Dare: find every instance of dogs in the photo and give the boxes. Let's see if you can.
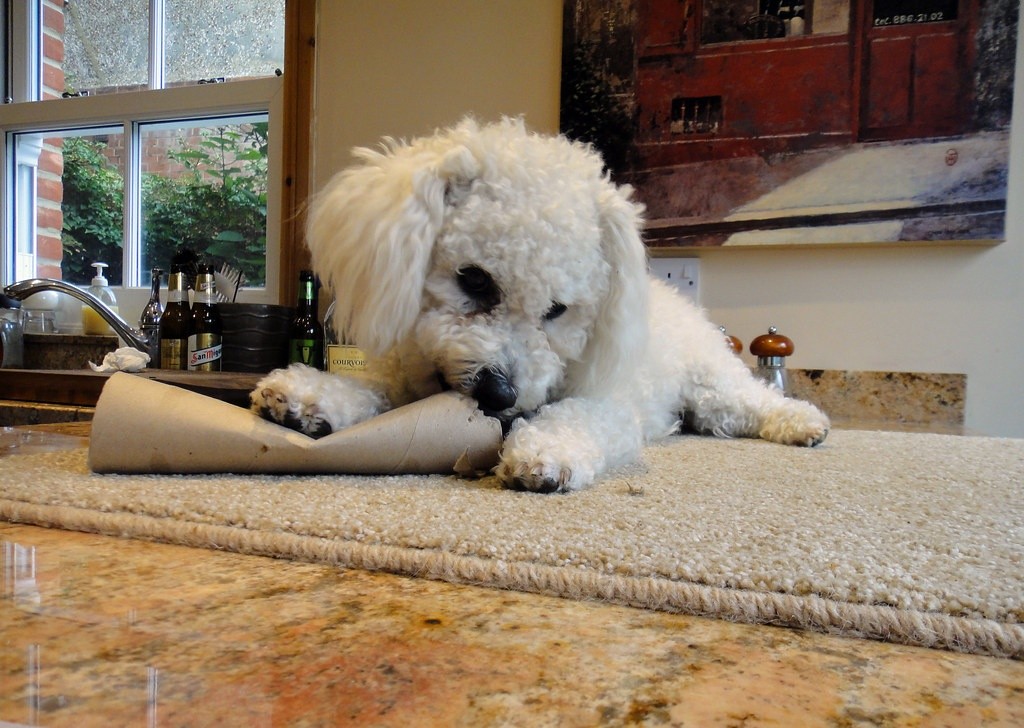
[249,112,828,493]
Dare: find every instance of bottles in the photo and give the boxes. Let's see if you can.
[160,264,190,373]
[720,326,742,356]
[187,265,223,372]
[747,327,794,391]
[292,271,322,368]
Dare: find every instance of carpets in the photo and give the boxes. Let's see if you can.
[0,428,1024,662]
